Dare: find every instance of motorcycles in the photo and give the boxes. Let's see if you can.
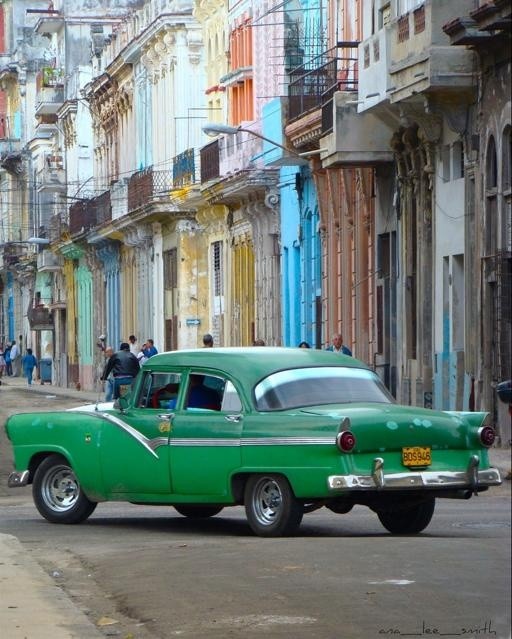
[99,371,139,401]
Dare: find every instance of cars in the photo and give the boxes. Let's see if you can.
[1,344,502,537]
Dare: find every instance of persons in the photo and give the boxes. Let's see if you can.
[299,342,309,348]
[254,340,265,346]
[99,336,158,402]
[167,374,220,409]
[326,333,352,355]
[20,348,38,387]
[203,334,213,348]
[0,335,23,385]
